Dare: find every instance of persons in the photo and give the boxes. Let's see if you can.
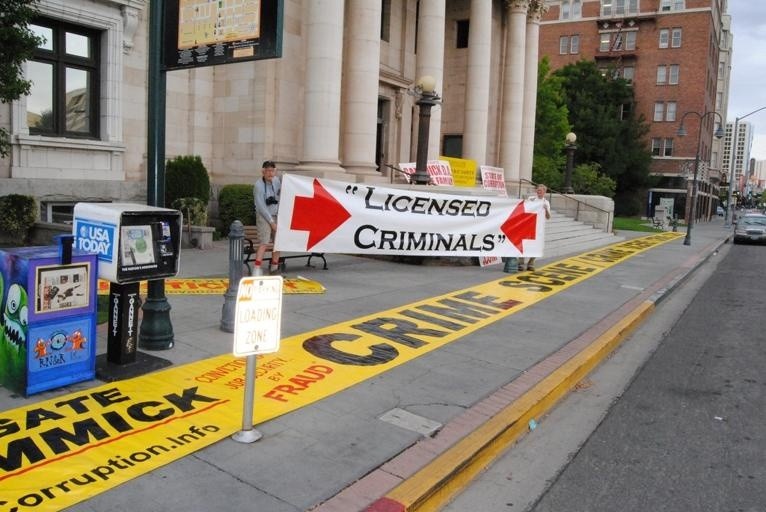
[518,184,551,270]
[252,160,282,278]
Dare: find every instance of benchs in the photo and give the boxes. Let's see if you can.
[241,223,328,277]
[651,216,665,231]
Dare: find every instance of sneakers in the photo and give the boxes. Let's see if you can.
[518,262,535,271]
[269,267,288,278]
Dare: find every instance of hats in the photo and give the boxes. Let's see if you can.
[262,160,276,168]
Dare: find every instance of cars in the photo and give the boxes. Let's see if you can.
[717,206,765,245]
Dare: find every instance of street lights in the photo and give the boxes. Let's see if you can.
[413,75,443,185]
[560,132,578,194]
[677,111,724,246]
